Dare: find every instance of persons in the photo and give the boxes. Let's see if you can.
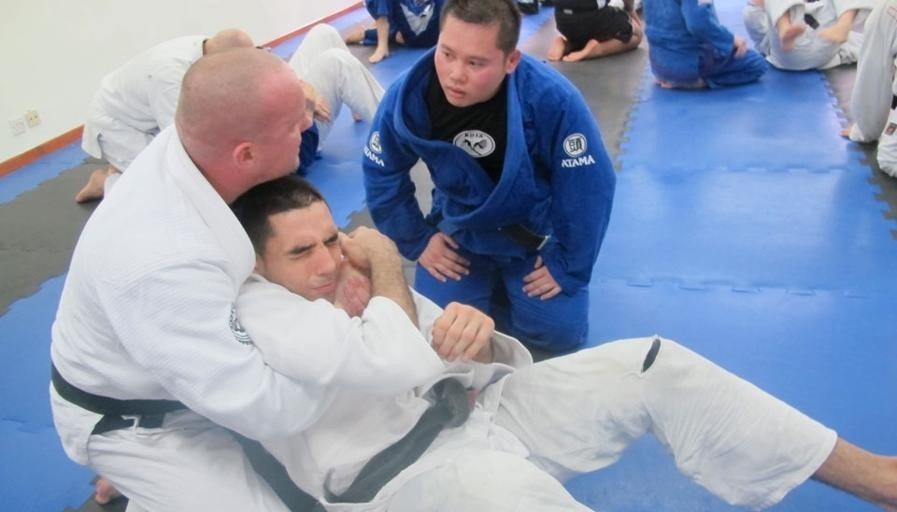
[44,47,382,511]
[360,2,619,360]
[545,1,643,64]
[342,1,442,65]
[72,26,251,204]
[212,172,896,512]
[740,0,876,74]
[836,0,896,179]
[635,0,768,90]
[280,19,387,184]
